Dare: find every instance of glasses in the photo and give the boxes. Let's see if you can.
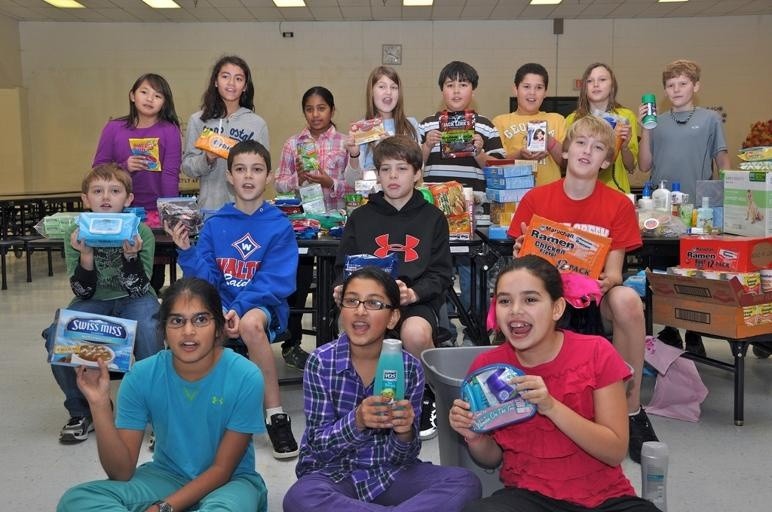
[340,296,394,310]
[163,313,215,329]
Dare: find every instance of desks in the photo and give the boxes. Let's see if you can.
[1,187,771,430]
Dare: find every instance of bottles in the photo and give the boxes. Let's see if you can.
[641,94,657,130]
[698,196,713,231]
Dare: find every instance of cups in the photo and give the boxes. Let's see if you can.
[669,180,694,228]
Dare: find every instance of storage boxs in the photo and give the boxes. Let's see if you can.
[718,168,771,240]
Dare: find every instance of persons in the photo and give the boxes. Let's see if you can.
[92,74,182,297]
[41,163,166,449]
[745,190,764,223]
[55,276,268,512]
[637,60,730,358]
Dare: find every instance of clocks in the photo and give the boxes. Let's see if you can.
[380,42,403,66]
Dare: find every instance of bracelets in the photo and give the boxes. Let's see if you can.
[152,500,173,512]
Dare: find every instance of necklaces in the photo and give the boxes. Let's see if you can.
[671,106,696,124]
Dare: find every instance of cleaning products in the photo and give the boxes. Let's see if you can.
[670,183,682,211]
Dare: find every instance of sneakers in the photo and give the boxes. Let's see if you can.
[280,344,309,371]
[420,394,437,440]
[59,416,92,441]
[265,412,299,458]
[657,328,707,357]
[627,408,659,463]
